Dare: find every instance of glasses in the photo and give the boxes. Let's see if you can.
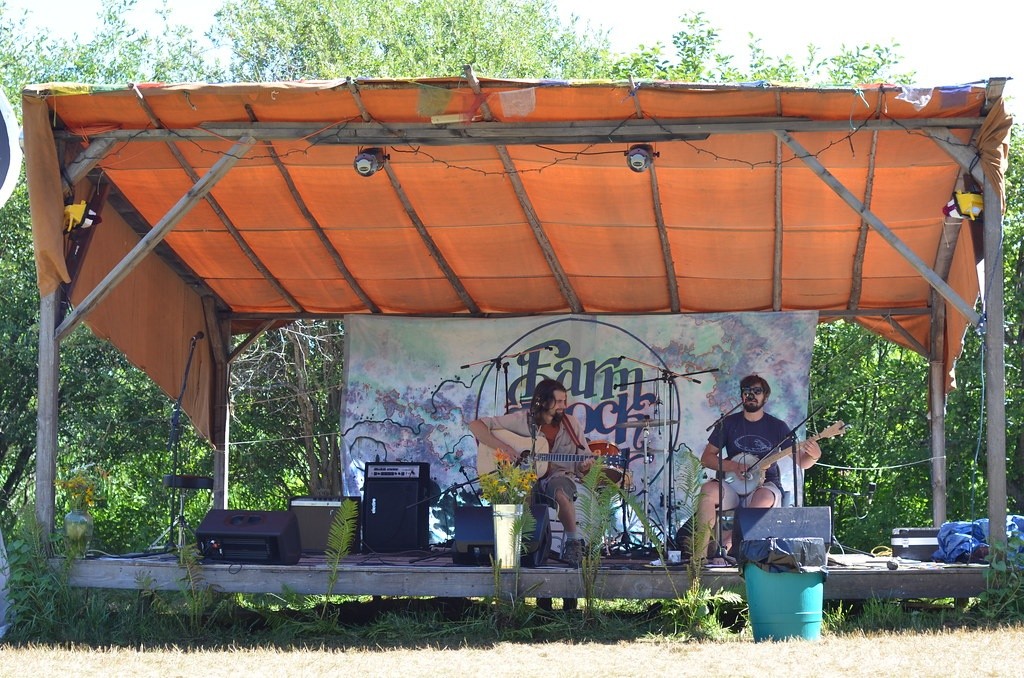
[742,387,764,395]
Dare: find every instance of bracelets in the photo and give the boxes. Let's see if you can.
[809,456,819,462]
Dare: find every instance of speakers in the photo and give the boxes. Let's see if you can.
[452,504,552,568]
[361,478,431,558]
[289,496,362,556]
[195,508,302,567]
[732,506,831,564]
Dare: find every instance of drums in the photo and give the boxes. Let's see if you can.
[579,439,623,486]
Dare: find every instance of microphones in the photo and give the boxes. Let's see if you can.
[191,331,204,341]
[533,396,544,410]
[740,471,753,481]
[746,393,755,401]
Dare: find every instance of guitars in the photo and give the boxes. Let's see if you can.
[475,428,628,473]
[725,419,853,498]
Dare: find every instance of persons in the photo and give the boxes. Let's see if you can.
[468,378,598,567]
[687,375,821,567]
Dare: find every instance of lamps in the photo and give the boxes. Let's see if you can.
[351,145,386,176]
[625,143,656,172]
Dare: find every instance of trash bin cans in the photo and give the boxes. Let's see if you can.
[742,538,826,643]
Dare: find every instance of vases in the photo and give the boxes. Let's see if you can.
[492,503,526,569]
[59,511,93,557]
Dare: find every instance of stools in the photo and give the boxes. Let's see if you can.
[714,510,734,556]
[548,491,580,559]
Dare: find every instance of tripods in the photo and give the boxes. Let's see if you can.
[88,337,200,562]
[599,448,649,553]
[665,399,749,566]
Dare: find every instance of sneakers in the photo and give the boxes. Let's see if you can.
[565,539,582,566]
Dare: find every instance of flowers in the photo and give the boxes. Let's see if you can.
[478,448,538,505]
[54,470,107,511]
